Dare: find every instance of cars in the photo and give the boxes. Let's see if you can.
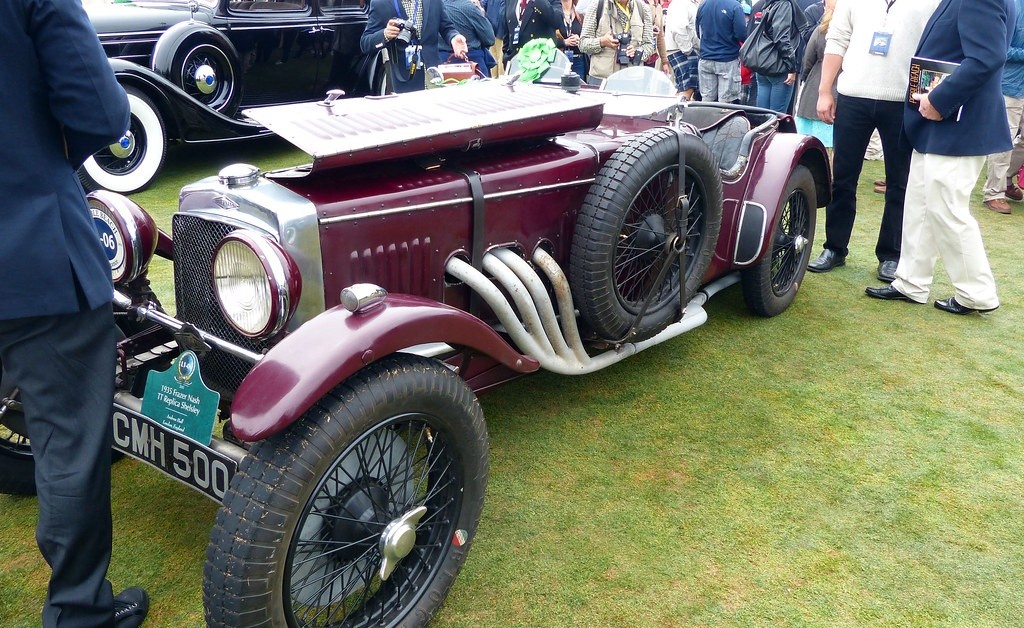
[2,65,840,628]
[69,0,395,196]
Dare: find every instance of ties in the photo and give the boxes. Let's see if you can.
[520,0,526,21]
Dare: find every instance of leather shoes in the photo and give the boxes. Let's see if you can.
[934,296,998,315]
[806,248,845,273]
[865,284,923,304]
[877,259,898,282]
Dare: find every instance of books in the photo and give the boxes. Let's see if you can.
[906,57,964,122]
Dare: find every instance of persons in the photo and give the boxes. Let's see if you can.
[0,0,149,628]
[359,0,1024,315]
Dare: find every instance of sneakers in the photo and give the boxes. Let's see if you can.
[1005,184,1022,201]
[111,586,148,628]
[985,199,1011,214]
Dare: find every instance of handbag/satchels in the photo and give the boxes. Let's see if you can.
[738,41,753,84]
[437,50,480,84]
[739,0,796,76]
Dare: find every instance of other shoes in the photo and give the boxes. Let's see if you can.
[874,186,886,194]
[873,180,887,185]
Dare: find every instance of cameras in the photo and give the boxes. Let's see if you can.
[395,18,417,51]
[613,33,631,66]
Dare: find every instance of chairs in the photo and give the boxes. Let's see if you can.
[672,95,748,169]
[230,1,302,9]
